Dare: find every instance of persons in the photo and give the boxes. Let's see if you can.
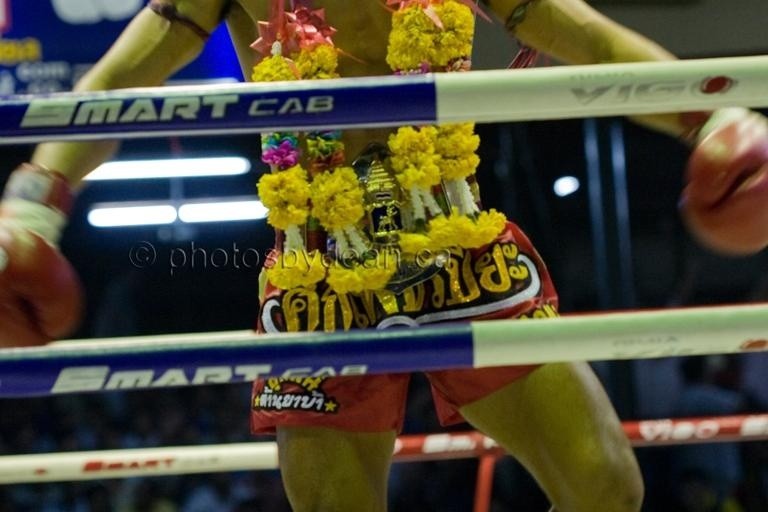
[0,0,768,512]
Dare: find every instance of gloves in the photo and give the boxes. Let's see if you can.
[675,108,767,258]
[0,162,88,351]
